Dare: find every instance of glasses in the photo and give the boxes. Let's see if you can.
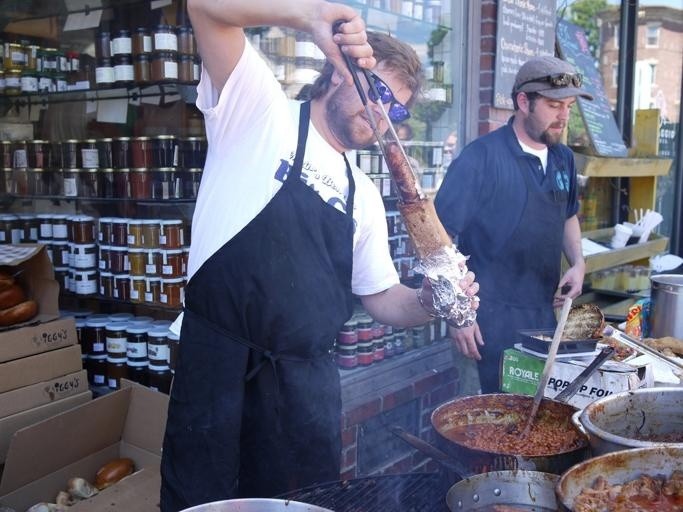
[515,69,585,92]
[353,61,412,126]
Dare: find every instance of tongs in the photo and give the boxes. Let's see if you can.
[601,324,683,376]
[332,17,427,207]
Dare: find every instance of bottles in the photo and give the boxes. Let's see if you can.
[0,212,191,396]
[331,138,449,371]
[0,135,205,200]
[574,193,600,233]
[0,41,96,95]
[243,0,455,110]
[92,25,204,86]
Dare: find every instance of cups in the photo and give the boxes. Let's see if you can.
[623,221,645,246]
[609,223,631,249]
[590,262,658,293]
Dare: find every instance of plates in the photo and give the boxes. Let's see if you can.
[516,329,601,352]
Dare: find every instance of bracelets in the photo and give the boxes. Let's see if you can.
[414,286,445,324]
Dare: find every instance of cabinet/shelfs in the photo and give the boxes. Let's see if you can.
[555,143,676,327]
[1,0,462,397]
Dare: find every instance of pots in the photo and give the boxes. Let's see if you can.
[555,442,683,512]
[427,346,618,475]
[571,385,683,456]
[649,273,682,343]
[391,424,563,512]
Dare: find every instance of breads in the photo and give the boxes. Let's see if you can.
[27,457,134,512]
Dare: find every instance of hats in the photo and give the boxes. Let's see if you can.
[511,54,595,103]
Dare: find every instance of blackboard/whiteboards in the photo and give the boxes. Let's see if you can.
[493,0,556,111]
[556,16,629,157]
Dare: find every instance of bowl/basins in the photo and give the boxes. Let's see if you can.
[179,497,339,511]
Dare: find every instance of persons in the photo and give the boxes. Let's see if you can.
[432,53,594,395]
[159,0,481,512]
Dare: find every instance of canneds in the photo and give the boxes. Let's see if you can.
[0,25,201,94]
[60,311,180,393]
[1,212,190,309]
[1,134,207,200]
[244,0,454,369]
